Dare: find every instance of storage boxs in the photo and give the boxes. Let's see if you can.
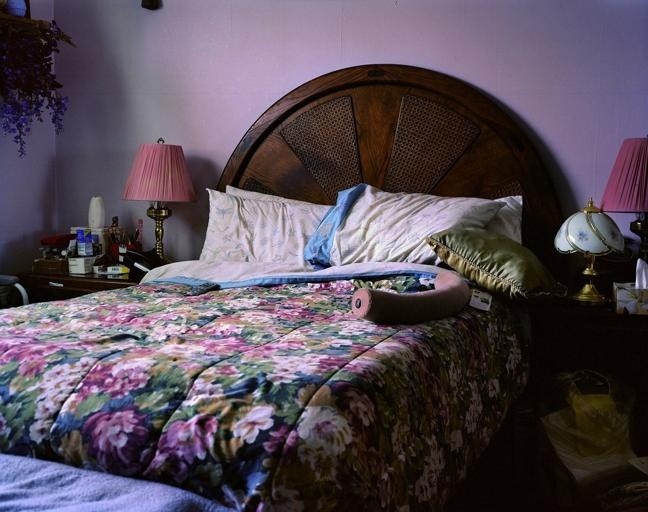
[69,226,121,257]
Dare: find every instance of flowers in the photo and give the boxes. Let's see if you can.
[0,14,82,162]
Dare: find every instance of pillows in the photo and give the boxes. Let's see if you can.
[486,191,527,247]
[304,180,506,270]
[204,186,332,275]
[424,221,555,302]
[223,183,313,205]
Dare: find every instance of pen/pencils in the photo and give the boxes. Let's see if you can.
[112,229,140,243]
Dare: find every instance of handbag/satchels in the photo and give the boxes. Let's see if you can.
[540,370,637,491]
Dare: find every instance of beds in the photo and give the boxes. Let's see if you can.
[1,62,580,511]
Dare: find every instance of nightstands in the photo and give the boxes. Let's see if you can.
[534,271,648,454]
[20,257,139,304]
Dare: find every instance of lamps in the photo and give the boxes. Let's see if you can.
[599,135,648,270]
[120,135,200,270]
[551,198,628,299]
